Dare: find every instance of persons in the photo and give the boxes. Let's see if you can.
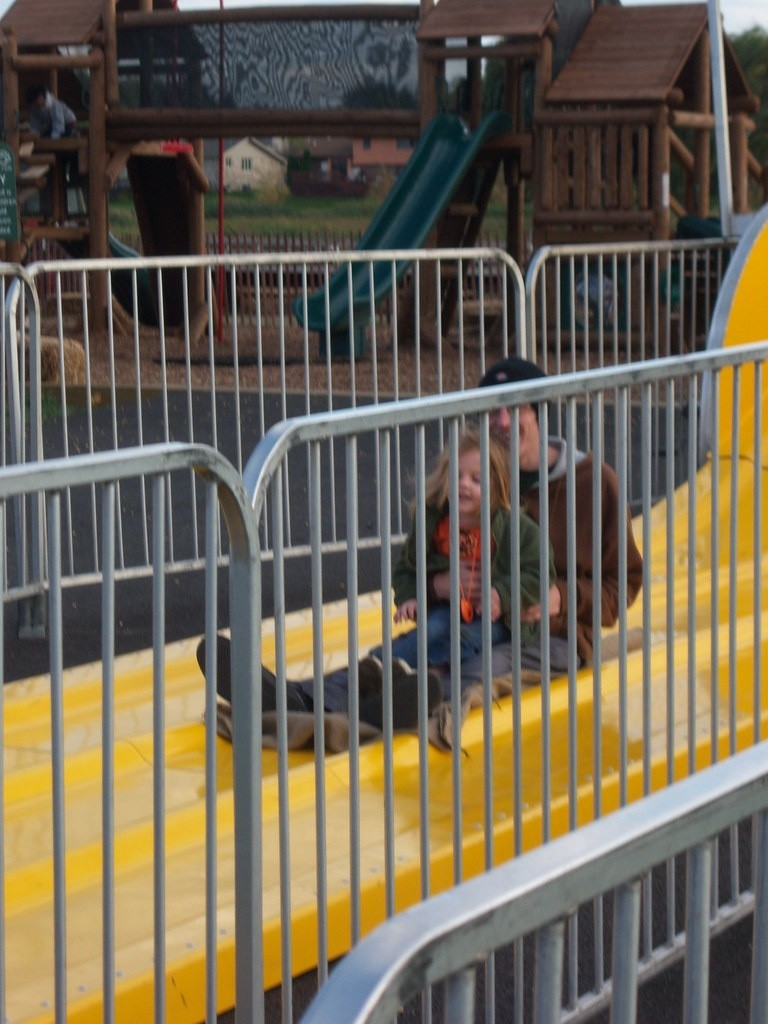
[196,356,642,731]
[25,85,77,140]
[675,216,731,335]
[358,426,558,723]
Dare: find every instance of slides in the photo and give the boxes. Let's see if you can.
[126,134,212,338]
[295,107,503,361]
[2,451,768,1024]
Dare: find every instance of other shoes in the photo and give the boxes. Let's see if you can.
[391,656,414,677]
[359,669,441,727]
[364,656,382,679]
[196,635,314,713]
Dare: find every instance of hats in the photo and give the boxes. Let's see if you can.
[479,357,550,422]
